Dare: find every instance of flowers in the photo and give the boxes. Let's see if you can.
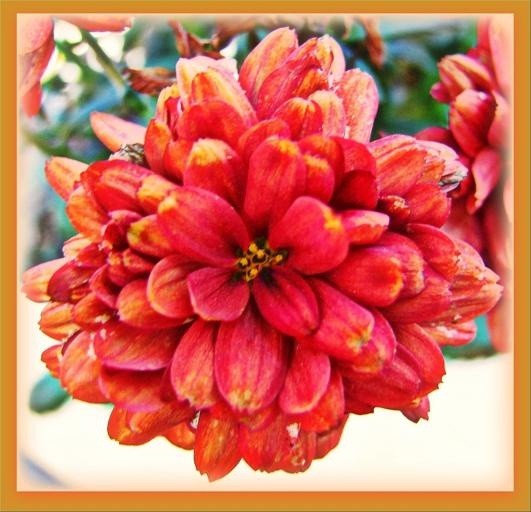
[16,13,516,493]
